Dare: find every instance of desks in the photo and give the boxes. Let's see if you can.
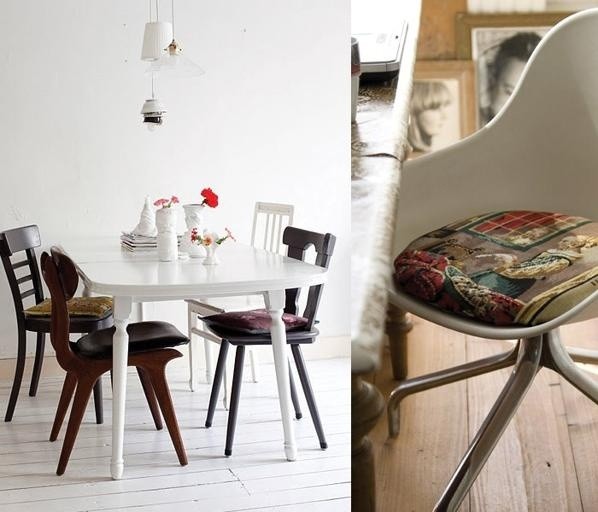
[349,0,424,512]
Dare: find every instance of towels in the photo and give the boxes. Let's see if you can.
[132,196,157,237]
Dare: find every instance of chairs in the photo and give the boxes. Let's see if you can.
[196,225,336,457]
[41,246,190,477]
[184,201,294,412]
[0,225,113,425]
[390,6,597,510]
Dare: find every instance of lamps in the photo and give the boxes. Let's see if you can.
[141,0,181,132]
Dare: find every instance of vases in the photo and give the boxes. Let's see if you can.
[156,209,178,262]
[181,204,204,250]
[202,245,219,265]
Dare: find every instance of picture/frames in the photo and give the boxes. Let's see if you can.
[457,10,577,139]
[397,61,478,164]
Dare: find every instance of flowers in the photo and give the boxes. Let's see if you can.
[200,187,218,208]
[190,227,238,245]
[154,196,179,207]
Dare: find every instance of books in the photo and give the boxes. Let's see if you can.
[121,234,182,251]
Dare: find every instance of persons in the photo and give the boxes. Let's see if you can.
[479,32,543,120]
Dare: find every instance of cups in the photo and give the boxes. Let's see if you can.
[184,204,206,231]
